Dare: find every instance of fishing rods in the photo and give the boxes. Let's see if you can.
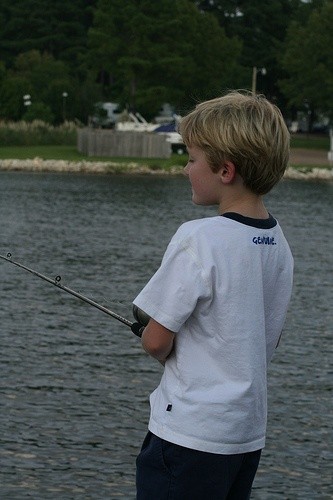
[0,252,143,336]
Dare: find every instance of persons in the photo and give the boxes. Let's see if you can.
[133,89,294,500]
